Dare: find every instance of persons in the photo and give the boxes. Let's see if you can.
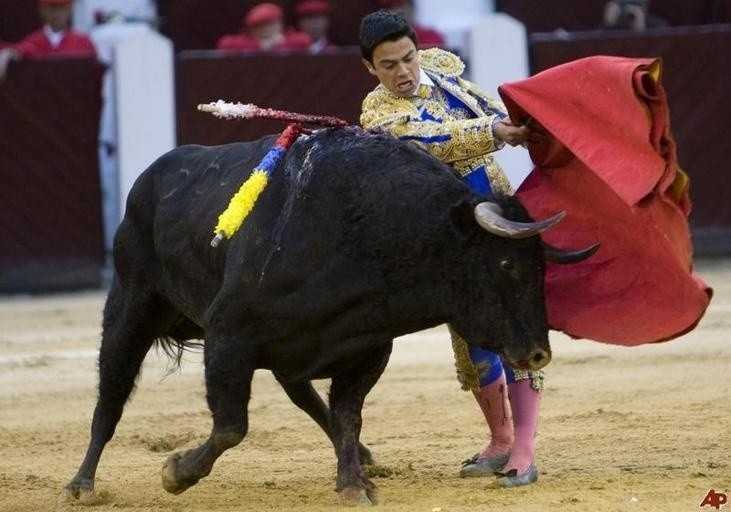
[596,0,672,30]
[359,9,546,489]
[217,3,313,50]
[0,0,95,58]
[294,0,337,49]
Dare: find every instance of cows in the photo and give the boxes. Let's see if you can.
[63,124,603,507]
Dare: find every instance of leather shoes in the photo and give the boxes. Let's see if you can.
[460,448,511,477]
[485,462,538,487]
